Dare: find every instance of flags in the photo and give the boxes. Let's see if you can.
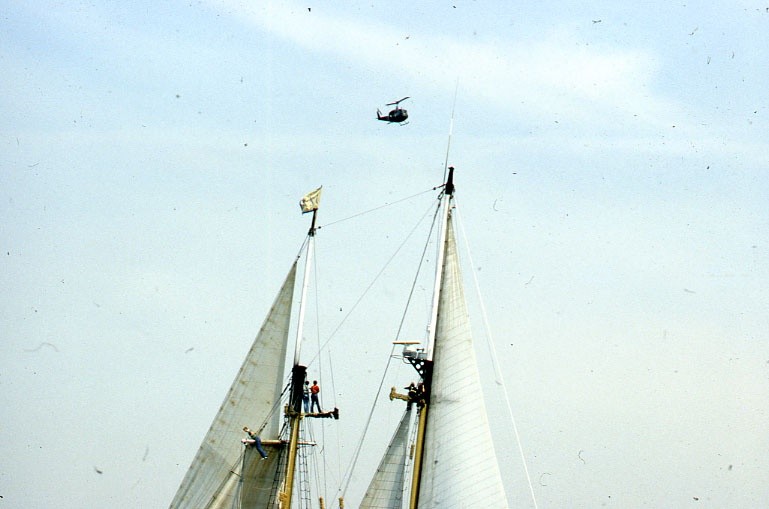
[300,187,321,213]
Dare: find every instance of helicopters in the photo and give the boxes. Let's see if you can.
[375,96,411,126]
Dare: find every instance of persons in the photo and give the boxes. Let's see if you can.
[243,427,268,461]
[302,381,310,414]
[310,380,323,413]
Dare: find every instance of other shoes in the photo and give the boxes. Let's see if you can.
[261,456,268,460]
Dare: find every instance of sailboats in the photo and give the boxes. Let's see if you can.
[167,167,541,509]
